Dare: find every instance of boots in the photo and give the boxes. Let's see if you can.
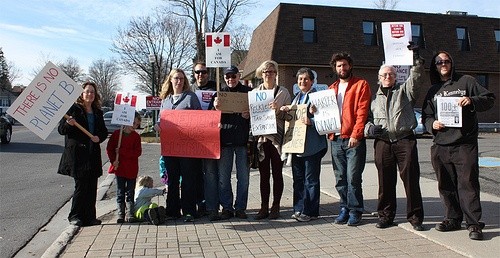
[255,202,268,219]
[269,202,280,219]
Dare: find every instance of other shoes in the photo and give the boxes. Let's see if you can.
[145,208,159,225]
[127,215,135,222]
[70,219,82,226]
[117,215,124,223]
[158,206,165,224]
[91,219,101,225]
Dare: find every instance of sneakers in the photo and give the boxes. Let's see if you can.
[347,214,362,225]
[296,213,317,222]
[468,225,483,240]
[236,210,247,218]
[335,213,347,223]
[435,219,461,231]
[184,214,194,222]
[292,212,301,218]
[208,209,219,220]
[220,210,234,219]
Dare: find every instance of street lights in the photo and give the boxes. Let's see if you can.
[148,51,156,133]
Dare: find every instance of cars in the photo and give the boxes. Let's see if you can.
[102,110,120,134]
[415,109,428,139]
[0,115,12,144]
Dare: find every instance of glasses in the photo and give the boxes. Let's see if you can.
[435,60,451,64]
[225,75,239,79]
[194,70,208,74]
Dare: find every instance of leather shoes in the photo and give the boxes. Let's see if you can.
[377,221,386,228]
[415,225,424,230]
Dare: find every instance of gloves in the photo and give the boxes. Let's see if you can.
[407,41,420,58]
[368,125,385,136]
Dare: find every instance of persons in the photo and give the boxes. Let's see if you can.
[134,176,165,225]
[251,60,291,219]
[307,52,371,226]
[106,109,142,223]
[159,153,183,200]
[422,51,496,240]
[180,62,218,219]
[208,65,254,220]
[455,116,459,124]
[280,67,328,222]
[57,82,108,225]
[364,40,426,231]
[153,68,203,222]
